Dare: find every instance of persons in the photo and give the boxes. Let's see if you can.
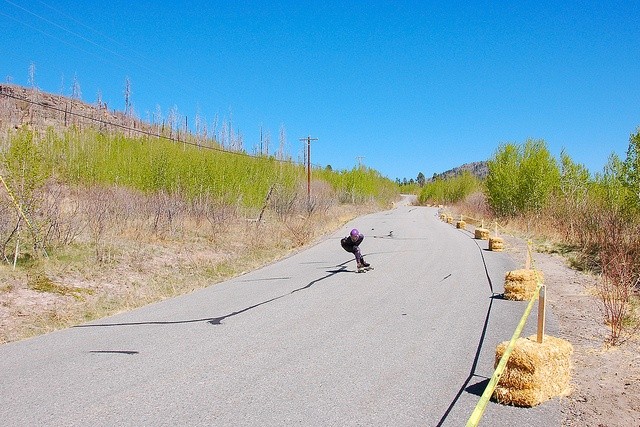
[341,229,370,268]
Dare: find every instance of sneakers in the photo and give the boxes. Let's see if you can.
[357,263,363,268]
[363,263,370,267]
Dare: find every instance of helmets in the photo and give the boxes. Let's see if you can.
[349,229,360,238]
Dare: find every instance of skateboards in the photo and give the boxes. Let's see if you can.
[355,267,373,273]
